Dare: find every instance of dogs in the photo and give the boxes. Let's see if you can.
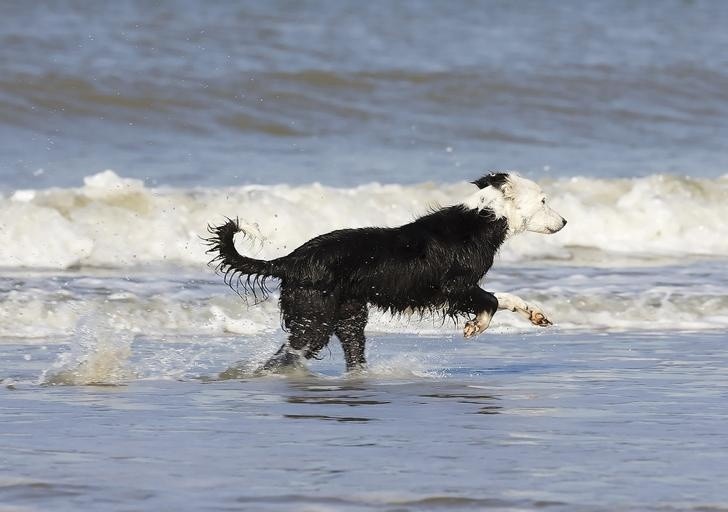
[198,169,567,373]
